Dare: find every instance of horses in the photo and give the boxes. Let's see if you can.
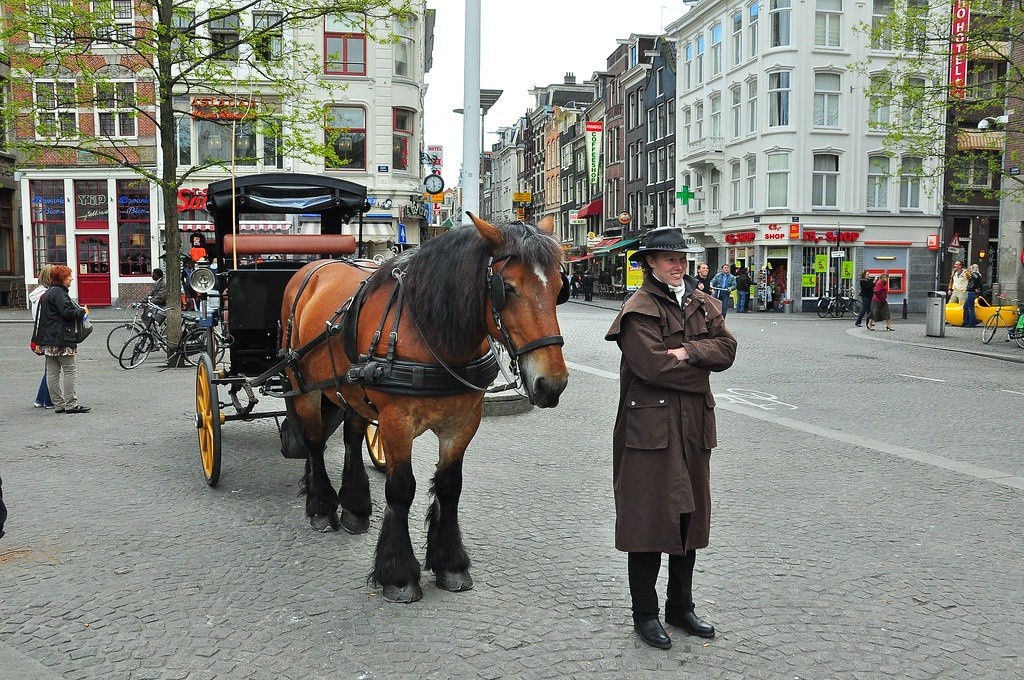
[281,209,572,602]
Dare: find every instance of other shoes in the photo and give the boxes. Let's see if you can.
[855,322,862,327]
[55,408,66,413]
[34,401,55,409]
[960,323,976,328]
[737,310,751,313]
[66,405,91,413]
[151,348,159,352]
[866,324,875,326]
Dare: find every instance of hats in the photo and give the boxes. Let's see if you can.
[628,226,705,261]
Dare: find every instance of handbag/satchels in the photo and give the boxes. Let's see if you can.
[30,336,46,355]
[76,320,93,343]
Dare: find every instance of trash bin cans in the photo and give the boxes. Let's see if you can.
[924,290,947,336]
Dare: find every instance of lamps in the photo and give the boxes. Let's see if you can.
[977,115,1009,131]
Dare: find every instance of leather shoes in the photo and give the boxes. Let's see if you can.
[634,618,672,649]
[665,610,715,638]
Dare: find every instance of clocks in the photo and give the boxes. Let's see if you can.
[424,174,445,194]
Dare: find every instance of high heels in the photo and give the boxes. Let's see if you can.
[867,325,875,331]
[886,326,895,331]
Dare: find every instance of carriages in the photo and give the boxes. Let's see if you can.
[193,173,569,603]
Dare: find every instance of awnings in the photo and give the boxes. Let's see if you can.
[562,237,639,263]
[578,199,602,219]
[341,223,396,243]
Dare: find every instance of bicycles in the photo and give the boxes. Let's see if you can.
[982,292,1024,348]
[712,288,728,299]
[106,300,225,369]
[817,285,865,319]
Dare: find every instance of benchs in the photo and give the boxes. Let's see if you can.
[220,232,356,371]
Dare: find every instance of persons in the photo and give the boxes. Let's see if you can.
[29,264,91,414]
[565,269,595,302]
[945,260,971,326]
[867,274,895,331]
[140,269,166,352]
[694,263,759,320]
[605,226,738,649]
[855,271,878,327]
[959,264,982,328]
[181,254,199,311]
